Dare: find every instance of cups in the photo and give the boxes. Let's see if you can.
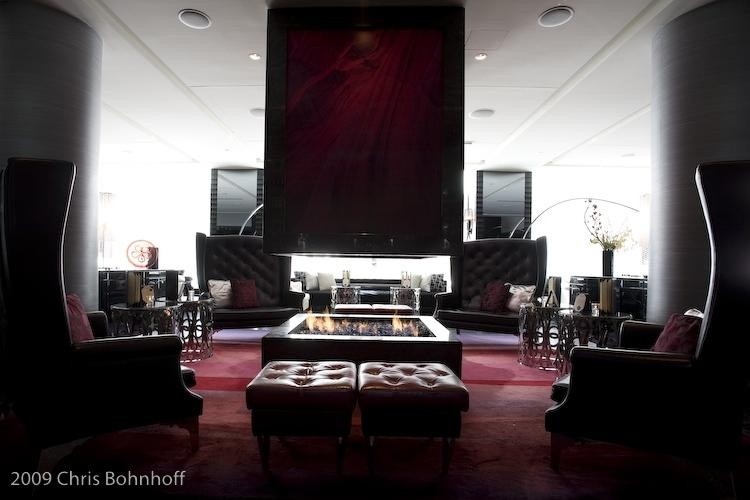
[181,282,195,300]
[591,302,600,317]
[543,296,550,308]
[148,247,158,269]
[147,295,155,309]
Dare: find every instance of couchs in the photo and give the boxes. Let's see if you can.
[282,273,448,317]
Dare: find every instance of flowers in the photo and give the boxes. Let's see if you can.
[576,193,634,250]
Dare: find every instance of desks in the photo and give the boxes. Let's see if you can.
[107,299,185,369]
[328,285,362,309]
[552,307,633,377]
[388,285,422,316]
[514,297,572,371]
[153,290,215,364]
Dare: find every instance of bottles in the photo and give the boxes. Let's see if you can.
[341,270,350,287]
[401,271,411,288]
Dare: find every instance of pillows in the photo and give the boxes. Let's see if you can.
[478,279,510,313]
[410,273,447,293]
[206,278,234,310]
[503,281,539,315]
[60,289,99,345]
[293,269,337,294]
[645,311,703,357]
[227,276,262,310]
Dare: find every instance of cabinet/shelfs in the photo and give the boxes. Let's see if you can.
[94,263,183,312]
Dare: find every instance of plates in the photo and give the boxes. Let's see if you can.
[125,240,157,268]
[572,293,587,313]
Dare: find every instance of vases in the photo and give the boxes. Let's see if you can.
[597,245,617,279]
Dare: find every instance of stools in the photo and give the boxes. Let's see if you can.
[354,358,470,482]
[241,355,357,477]
[370,302,415,317]
[332,302,372,314]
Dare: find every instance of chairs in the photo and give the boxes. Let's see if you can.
[195,233,305,328]
[541,151,748,498]
[434,238,551,340]
[0,154,204,485]
